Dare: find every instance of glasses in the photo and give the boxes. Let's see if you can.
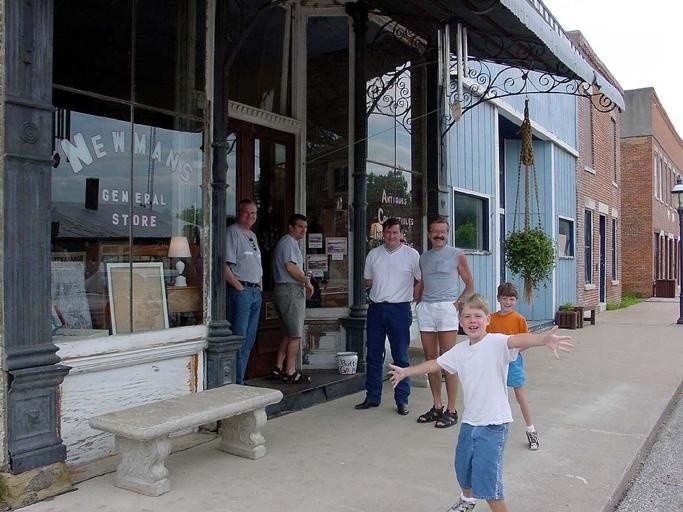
[249,238,256,251]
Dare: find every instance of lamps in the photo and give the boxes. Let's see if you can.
[166,235,194,287]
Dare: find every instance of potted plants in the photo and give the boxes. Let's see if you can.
[555,302,581,330]
[502,225,559,297]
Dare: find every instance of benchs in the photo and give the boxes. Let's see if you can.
[88,385,285,498]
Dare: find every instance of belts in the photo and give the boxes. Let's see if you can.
[239,281,260,288]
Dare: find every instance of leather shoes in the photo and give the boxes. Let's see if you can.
[397,403,409,415]
[355,399,378,409]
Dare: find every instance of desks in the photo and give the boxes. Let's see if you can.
[165,287,202,328]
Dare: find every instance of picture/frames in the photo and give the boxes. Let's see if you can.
[104,260,169,336]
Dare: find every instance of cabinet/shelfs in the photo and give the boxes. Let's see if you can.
[98,243,200,300]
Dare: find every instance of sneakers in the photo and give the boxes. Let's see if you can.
[446,498,476,512]
[526,431,540,450]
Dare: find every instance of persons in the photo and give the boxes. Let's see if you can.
[355,218,421,414]
[486,282,539,450]
[269,214,314,384]
[387,290,575,512]
[224,197,263,385]
[414,217,474,429]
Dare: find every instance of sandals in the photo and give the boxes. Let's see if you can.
[417,405,444,423]
[272,367,283,379]
[282,372,312,384]
[435,409,458,428]
